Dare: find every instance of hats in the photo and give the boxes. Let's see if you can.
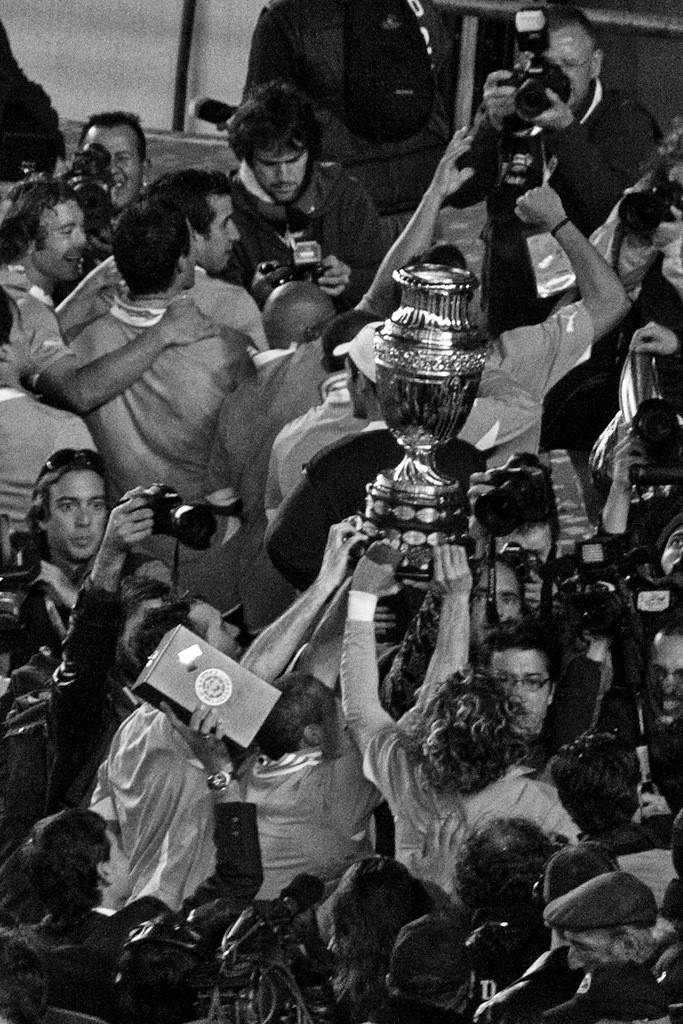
[331,320,383,385]
[542,871,657,933]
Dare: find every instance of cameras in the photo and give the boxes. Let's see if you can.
[476,452,555,536]
[502,6,570,122]
[134,482,215,552]
[630,400,682,484]
[619,183,683,234]
[56,143,113,222]
[275,873,324,918]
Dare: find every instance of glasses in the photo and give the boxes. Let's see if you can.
[495,674,552,693]
[38,448,103,478]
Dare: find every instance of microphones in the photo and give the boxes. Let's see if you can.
[283,209,319,284]
[190,96,235,127]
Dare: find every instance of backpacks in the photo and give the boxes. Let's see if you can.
[293,1,434,144]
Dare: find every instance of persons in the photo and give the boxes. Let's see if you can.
[4,0,683,1021]
